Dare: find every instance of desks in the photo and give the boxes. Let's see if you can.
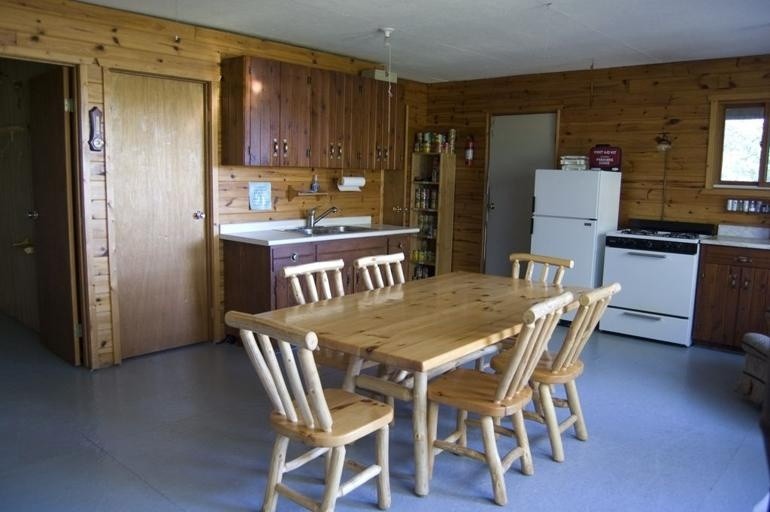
[225,269,596,498]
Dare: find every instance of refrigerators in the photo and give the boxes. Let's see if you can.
[529,168,622,328]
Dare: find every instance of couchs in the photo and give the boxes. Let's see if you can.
[736,332,770,410]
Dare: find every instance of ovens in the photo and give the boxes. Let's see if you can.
[600,246,700,348]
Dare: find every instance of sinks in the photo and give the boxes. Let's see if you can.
[293,227,345,236]
[323,224,374,233]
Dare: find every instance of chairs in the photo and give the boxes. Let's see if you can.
[281,257,399,426]
[225,308,397,511]
[490,280,621,463]
[354,253,457,381]
[475,253,576,395]
[426,291,574,506]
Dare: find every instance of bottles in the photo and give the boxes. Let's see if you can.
[415,188,437,209]
[413,214,436,262]
[414,126,458,154]
[727,199,769,214]
[309,175,320,192]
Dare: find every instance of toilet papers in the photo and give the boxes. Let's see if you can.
[336,176,366,192]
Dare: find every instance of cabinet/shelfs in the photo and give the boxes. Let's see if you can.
[409,151,457,281]
[310,67,351,169]
[220,54,311,168]
[388,233,412,286]
[224,240,315,341]
[350,74,402,171]
[315,236,389,300]
[691,245,769,350]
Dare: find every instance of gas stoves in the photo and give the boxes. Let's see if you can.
[603,225,702,254]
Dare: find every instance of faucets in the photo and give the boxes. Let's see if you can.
[305,204,337,228]
